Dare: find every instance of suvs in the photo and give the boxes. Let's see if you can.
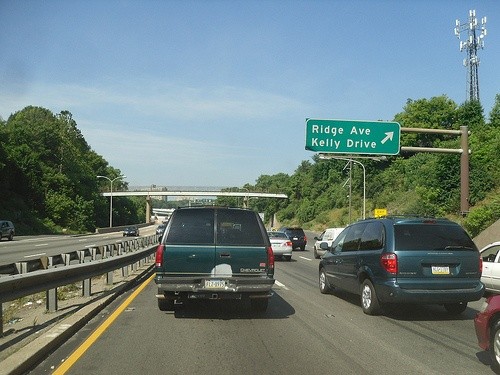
[153,205,276,313]
[0,221,15,241]
[277,227,307,251]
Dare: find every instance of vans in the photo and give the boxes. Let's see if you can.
[318,214,486,316]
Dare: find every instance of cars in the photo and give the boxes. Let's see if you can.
[478,241,500,296]
[155,217,168,235]
[313,227,345,259]
[474,294,500,372]
[123,225,139,237]
[267,230,293,261]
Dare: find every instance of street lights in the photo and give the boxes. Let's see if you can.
[96,175,127,228]
[318,156,367,220]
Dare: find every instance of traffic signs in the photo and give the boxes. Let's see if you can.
[304,117,401,157]
